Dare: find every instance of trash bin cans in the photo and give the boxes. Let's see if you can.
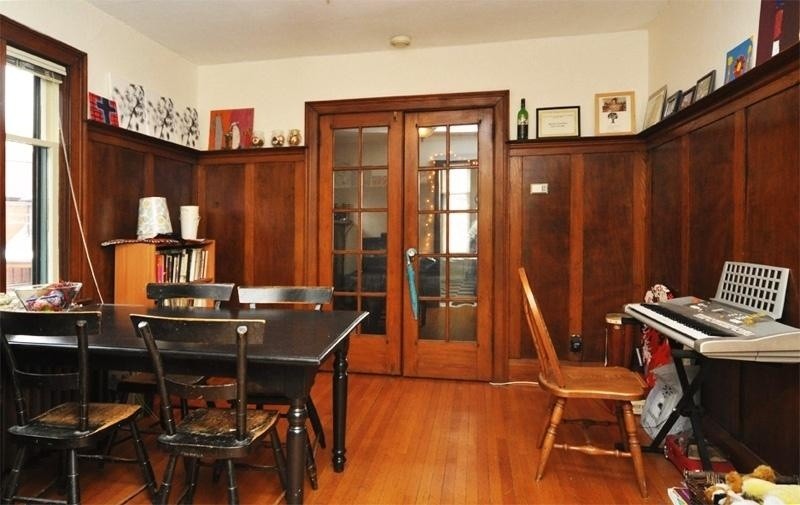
[605,312,637,369]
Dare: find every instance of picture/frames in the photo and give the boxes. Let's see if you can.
[722,37,756,85]
[642,86,664,123]
[595,91,634,136]
[536,105,579,137]
[661,91,682,122]
[677,86,695,110]
[690,71,717,103]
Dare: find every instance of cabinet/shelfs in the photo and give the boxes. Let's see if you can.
[502,142,647,386]
[85,120,196,305]
[109,239,215,322]
[638,41,800,486]
[198,147,305,310]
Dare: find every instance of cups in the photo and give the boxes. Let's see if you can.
[270,130,285,146]
[250,130,265,147]
[287,129,301,146]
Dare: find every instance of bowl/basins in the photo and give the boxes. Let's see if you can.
[11,281,84,312]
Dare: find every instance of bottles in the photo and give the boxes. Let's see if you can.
[516,98,528,141]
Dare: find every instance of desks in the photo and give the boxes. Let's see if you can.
[0,307,371,505]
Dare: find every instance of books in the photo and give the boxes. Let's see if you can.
[163,298,209,307]
[155,248,208,283]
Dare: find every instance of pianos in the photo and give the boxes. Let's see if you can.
[622,292,795,368]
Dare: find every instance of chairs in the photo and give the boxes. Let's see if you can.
[238,286,335,487]
[131,312,291,505]
[0,311,152,505]
[519,267,649,498]
[145,278,233,445]
